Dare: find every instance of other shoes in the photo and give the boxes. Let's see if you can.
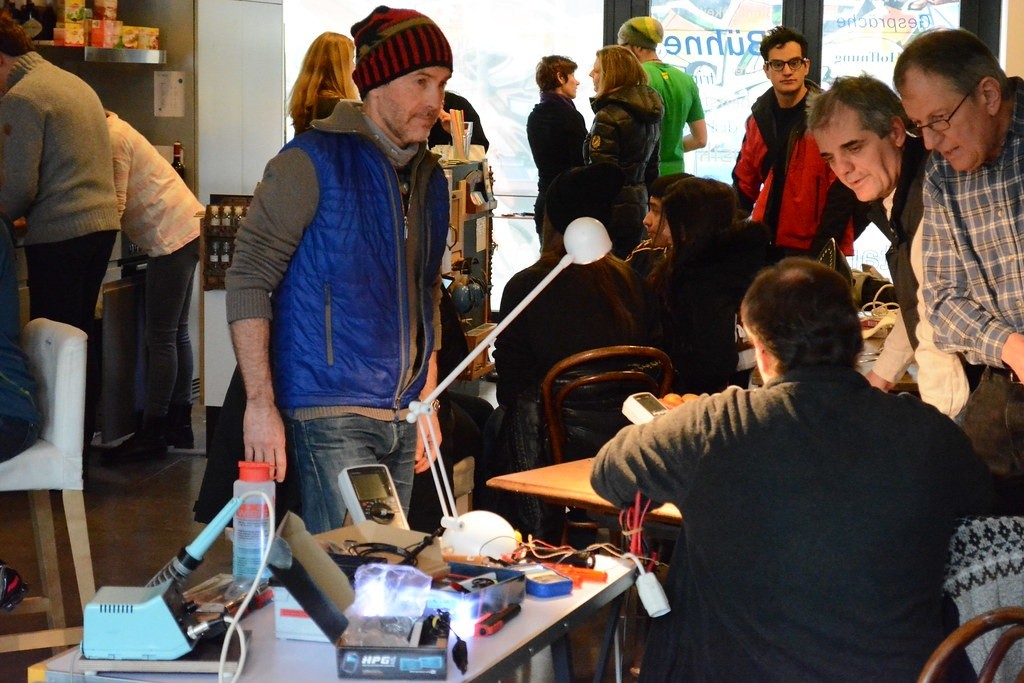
[103,433,166,466]
[164,424,194,450]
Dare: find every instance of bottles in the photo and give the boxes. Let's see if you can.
[232,460,278,581]
[208,205,250,268]
[171,143,185,179]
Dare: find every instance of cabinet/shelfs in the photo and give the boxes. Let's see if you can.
[200,194,254,458]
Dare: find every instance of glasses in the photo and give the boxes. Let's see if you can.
[905,92,971,138]
[765,58,807,71]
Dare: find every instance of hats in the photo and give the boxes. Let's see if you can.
[352,6,453,100]
[619,17,664,50]
[545,161,625,234]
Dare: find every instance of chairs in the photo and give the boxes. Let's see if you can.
[542,345,674,547]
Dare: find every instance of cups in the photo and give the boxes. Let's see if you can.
[435,144,452,166]
[450,121,474,163]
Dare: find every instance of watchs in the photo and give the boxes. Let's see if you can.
[421,398,440,410]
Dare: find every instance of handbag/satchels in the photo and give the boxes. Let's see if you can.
[858,283,900,341]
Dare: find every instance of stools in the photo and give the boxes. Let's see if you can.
[0,317,96,656]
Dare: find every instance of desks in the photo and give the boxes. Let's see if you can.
[27,524,640,683]
[486,456,682,553]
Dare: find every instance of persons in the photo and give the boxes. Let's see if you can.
[0,6,1024,683]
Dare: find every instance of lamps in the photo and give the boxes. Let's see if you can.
[405,217,613,560]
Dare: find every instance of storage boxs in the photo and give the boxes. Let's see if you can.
[266,510,525,681]
[53,0,160,50]
